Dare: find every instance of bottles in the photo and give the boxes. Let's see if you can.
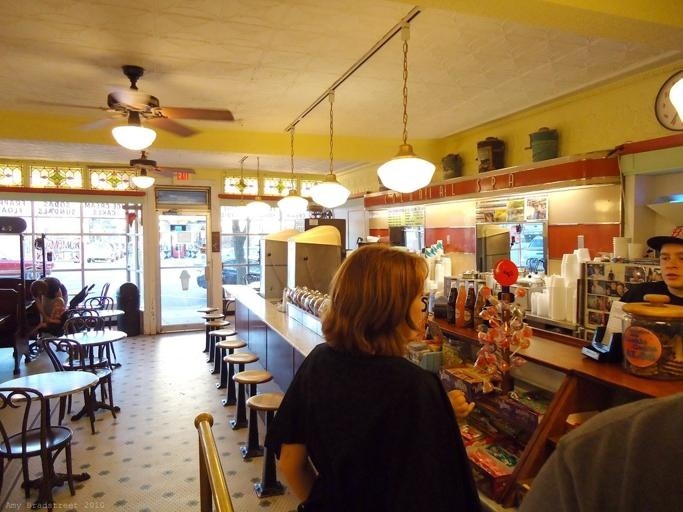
[474,285,486,330]
[447,281,459,326]
[455,282,466,329]
[463,284,476,329]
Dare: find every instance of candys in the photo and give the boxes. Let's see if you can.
[474,286,533,373]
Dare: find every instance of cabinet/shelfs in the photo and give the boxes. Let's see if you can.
[505,356,683,512]
[420,317,583,512]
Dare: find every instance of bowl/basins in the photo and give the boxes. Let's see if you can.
[611,236,643,258]
[531,248,590,323]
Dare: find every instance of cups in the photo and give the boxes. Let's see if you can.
[422,239,443,282]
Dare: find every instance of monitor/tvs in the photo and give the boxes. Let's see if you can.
[499,221,548,274]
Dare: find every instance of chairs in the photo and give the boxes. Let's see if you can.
[0,277,136,511]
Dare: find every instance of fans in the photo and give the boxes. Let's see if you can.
[13,64,235,140]
[86,145,197,178]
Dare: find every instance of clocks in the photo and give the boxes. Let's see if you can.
[652,68,683,134]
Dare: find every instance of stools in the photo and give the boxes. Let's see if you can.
[197,305,289,503]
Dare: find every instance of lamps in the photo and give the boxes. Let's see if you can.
[111,110,156,153]
[129,170,156,192]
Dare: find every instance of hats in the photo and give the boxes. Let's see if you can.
[647,226,683,249]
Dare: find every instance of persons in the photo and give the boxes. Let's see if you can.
[263,240,484,512]
[195,243,208,289]
[24,296,65,339]
[231,215,250,286]
[30,274,68,322]
[510,389,683,510]
[618,224,683,303]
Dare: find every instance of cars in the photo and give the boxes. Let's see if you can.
[0,257,55,280]
[196,256,259,290]
[84,239,133,264]
